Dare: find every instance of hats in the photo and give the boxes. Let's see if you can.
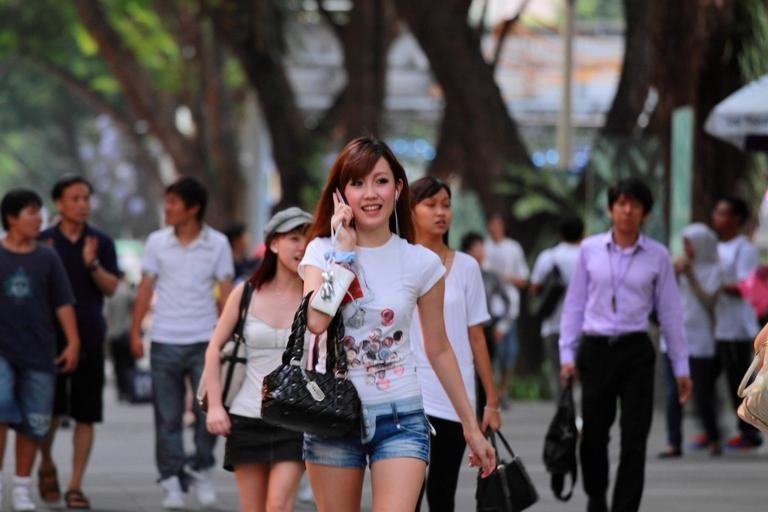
[263,207,314,246]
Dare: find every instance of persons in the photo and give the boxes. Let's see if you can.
[0,188,80,512]
[130,178,237,509]
[403,177,501,512]
[202,204,314,512]
[223,221,252,285]
[37,176,119,509]
[297,134,496,512]
[102,268,140,397]
[556,177,694,512]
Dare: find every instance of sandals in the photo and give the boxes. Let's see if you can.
[64,489,90,510]
[38,465,60,502]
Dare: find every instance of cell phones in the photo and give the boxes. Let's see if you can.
[394,189,399,199]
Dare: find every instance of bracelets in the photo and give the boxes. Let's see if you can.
[324,250,356,268]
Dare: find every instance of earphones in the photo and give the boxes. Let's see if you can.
[336,186,346,207]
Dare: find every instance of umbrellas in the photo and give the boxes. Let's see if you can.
[702,71,768,155]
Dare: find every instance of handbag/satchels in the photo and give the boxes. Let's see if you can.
[260,291,364,438]
[543,374,579,502]
[737,342,768,432]
[195,280,254,412]
[477,426,538,512]
[532,251,566,319]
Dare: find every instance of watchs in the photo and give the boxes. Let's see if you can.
[88,257,101,273]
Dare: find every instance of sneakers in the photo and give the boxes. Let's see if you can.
[161,477,186,509]
[656,432,761,457]
[11,485,35,510]
[190,473,218,506]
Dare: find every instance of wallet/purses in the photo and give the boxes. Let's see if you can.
[311,222,354,314]
